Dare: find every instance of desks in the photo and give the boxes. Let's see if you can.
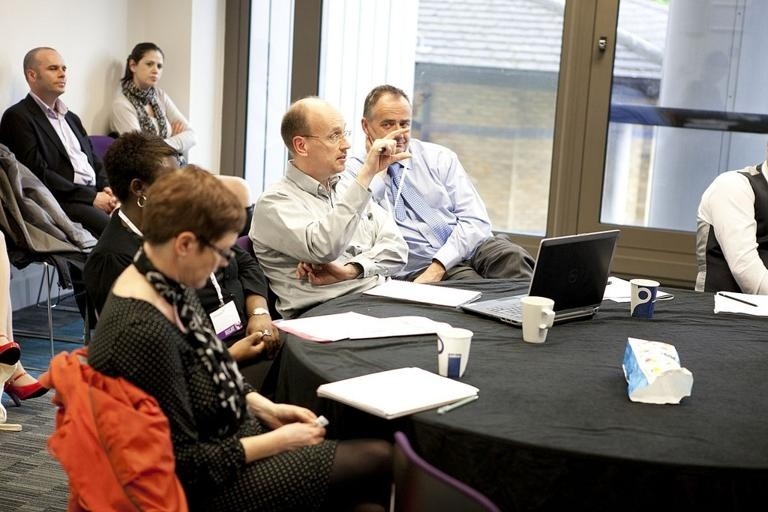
[285,280,768,512]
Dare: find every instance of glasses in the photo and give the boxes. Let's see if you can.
[176,229,236,264]
[292,129,351,144]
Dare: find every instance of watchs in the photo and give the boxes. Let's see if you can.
[246,306,270,319]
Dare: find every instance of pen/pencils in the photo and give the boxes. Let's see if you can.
[437,395,480,416]
[378,147,385,152]
[718,293,758,307]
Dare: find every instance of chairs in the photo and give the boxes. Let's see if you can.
[65,347,177,512]
[0,157,84,373]
[209,171,252,209]
[393,430,501,512]
[87,135,117,159]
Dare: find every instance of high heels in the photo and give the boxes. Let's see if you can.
[0,335,21,365]
[4,372,50,406]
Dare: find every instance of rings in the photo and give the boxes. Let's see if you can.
[257,327,270,339]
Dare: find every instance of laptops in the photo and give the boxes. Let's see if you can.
[461,230,621,327]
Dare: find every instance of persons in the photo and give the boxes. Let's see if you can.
[248,97,413,320]
[88,165,395,512]
[695,142,768,296]
[341,85,536,284]
[677,50,731,112]
[0,48,121,240]
[109,42,255,238]
[0,232,51,407]
[83,130,288,391]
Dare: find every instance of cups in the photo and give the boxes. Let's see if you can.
[629,278,660,318]
[437,327,474,379]
[520,296,555,344]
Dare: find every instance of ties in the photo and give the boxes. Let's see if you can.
[389,163,452,245]
